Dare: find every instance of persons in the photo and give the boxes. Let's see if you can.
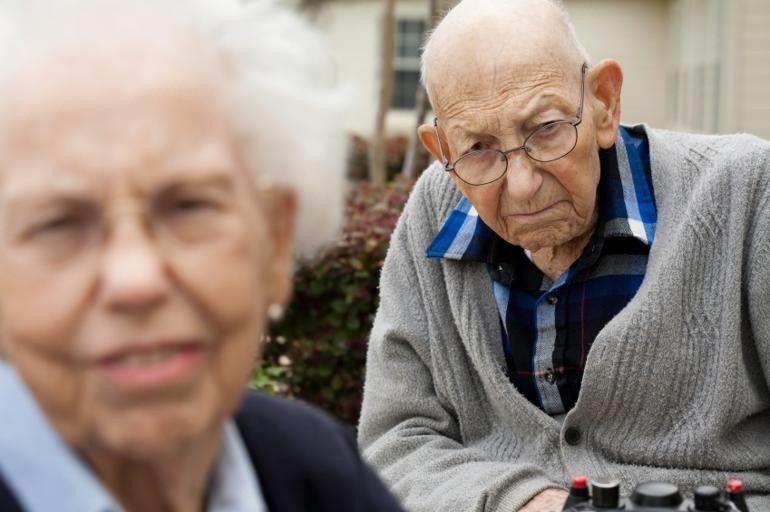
[0,2,405,511]
[358,1,770,510]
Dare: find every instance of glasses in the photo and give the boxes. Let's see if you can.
[433,63,589,187]
